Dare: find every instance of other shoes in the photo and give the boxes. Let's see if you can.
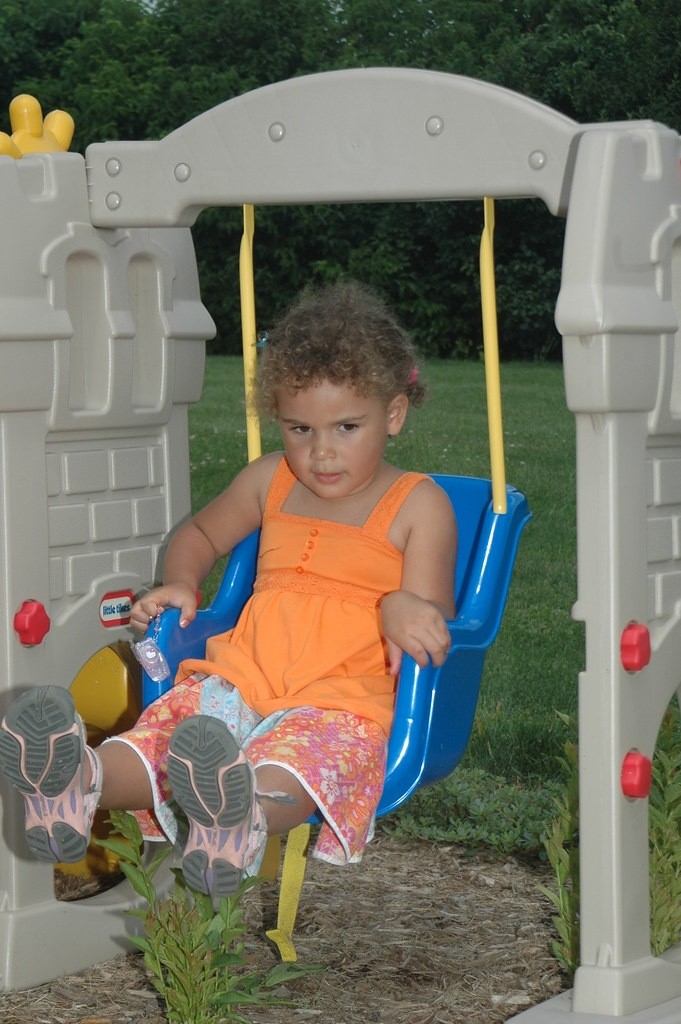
[0,685,91,864]
[167,715,253,897]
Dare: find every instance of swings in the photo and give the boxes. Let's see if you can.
[136,196,539,829]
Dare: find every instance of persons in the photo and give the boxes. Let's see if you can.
[0,284,457,892]
